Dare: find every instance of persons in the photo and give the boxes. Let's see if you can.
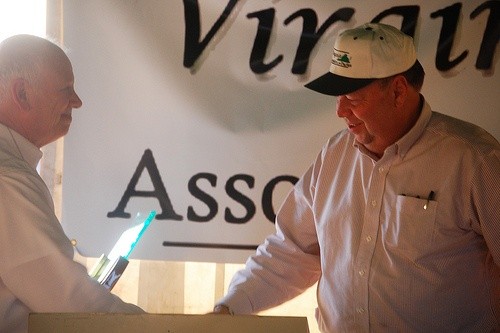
[213,21,500,332]
[0,35,146,332]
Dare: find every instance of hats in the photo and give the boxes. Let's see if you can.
[304,23,417,96]
